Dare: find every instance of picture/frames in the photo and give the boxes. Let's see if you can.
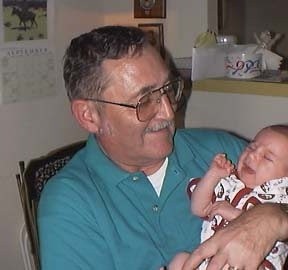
[137,24,164,48]
[134,0,166,19]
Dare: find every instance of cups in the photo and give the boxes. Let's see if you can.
[225,54,262,80]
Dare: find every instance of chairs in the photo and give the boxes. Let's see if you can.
[15,140,88,270]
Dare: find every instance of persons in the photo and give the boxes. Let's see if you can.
[37,26,251,270]
[167,124,288,270]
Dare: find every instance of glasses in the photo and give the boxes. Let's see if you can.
[85,76,184,122]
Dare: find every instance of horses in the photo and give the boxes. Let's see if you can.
[10,7,38,32]
